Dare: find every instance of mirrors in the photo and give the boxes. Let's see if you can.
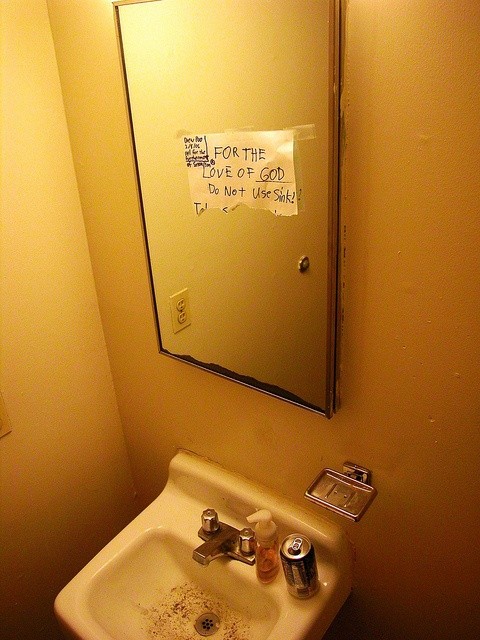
[112,1,344,419]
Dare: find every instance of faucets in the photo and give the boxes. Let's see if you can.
[190,507,257,567]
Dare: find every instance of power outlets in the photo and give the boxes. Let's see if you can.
[170,288,191,334]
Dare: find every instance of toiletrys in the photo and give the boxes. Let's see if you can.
[245,509,281,584]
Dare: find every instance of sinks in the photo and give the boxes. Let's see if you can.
[52,449,354,640]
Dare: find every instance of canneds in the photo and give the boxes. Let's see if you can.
[279,533,320,600]
